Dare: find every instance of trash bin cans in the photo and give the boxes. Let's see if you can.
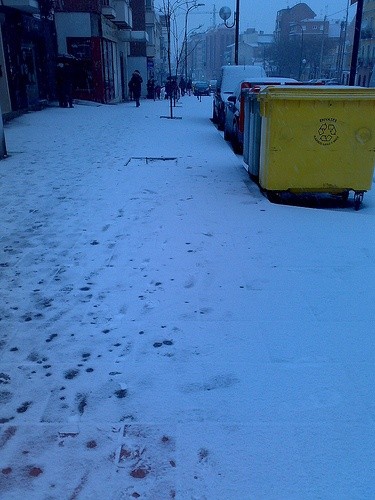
[242,84,374,210]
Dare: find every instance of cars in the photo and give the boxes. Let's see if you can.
[193,82,212,96]
[224,77,343,155]
[192,79,217,93]
[212,65,268,131]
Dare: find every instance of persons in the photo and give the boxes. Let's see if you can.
[147,75,193,101]
[56,62,74,109]
[129,69,143,107]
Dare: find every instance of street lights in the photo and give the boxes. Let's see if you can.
[290,23,305,82]
[184,3,205,83]
[218,5,239,65]
